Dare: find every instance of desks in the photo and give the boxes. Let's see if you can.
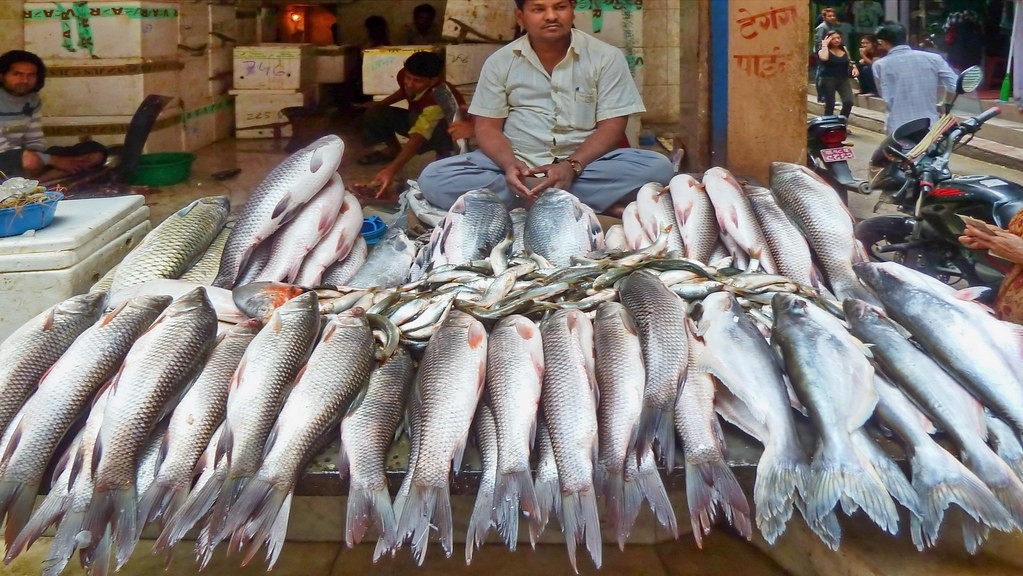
[31,429,960,499]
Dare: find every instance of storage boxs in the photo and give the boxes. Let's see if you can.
[0,0,644,157]
[0,196,153,348]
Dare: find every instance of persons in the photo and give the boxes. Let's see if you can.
[856,35,887,97]
[957,208,1023,325]
[0,50,107,184]
[416,0,674,219]
[814,7,837,103]
[868,20,959,211]
[362,15,390,48]
[350,49,475,200]
[816,30,859,134]
[409,3,440,45]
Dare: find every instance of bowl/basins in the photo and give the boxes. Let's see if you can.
[135,151,196,186]
[0,192,64,238]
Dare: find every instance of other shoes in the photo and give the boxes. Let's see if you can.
[358,150,395,165]
[847,128,851,134]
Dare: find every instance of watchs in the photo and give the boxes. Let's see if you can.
[565,157,584,176]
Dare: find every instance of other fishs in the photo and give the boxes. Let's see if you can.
[0,135,1023,576]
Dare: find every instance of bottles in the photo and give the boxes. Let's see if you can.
[1000,72,1010,101]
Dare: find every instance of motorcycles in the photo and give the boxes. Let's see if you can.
[806,114,873,208]
[849,65,1023,299]
